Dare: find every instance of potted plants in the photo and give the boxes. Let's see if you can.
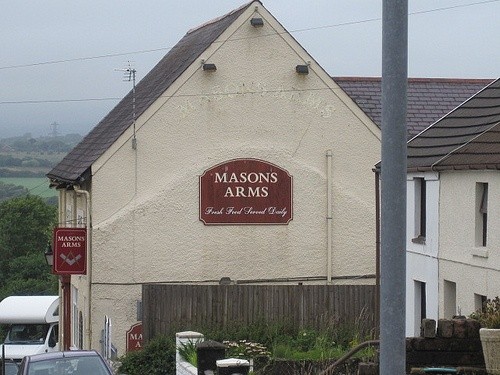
[469,296,500,375]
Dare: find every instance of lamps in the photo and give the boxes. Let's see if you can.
[48,183,58,189]
[56,183,67,190]
[296,65,308,74]
[203,64,217,71]
[220,277,231,284]
[251,17,263,26]
[45,217,86,267]
[66,185,74,190]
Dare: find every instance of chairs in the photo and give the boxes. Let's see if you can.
[13,331,29,341]
[77,357,104,375]
[31,367,55,375]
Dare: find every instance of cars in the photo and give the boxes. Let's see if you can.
[18,349,118,375]
[0,359,19,375]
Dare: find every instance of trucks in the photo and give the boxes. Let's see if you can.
[0,295,60,360]
